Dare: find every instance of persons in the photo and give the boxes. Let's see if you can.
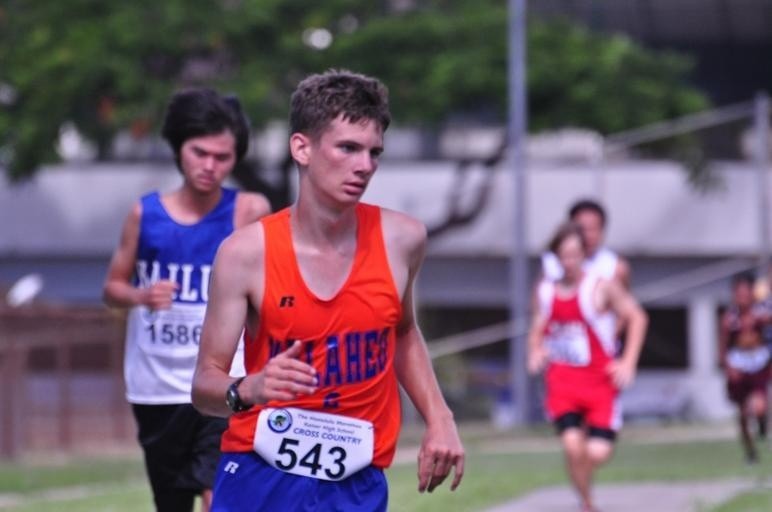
[715,271,772,465]
[529,199,632,355]
[523,220,650,512]
[190,66,466,512]
[99,85,272,511]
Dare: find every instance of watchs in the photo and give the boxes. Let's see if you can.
[225,374,254,413]
